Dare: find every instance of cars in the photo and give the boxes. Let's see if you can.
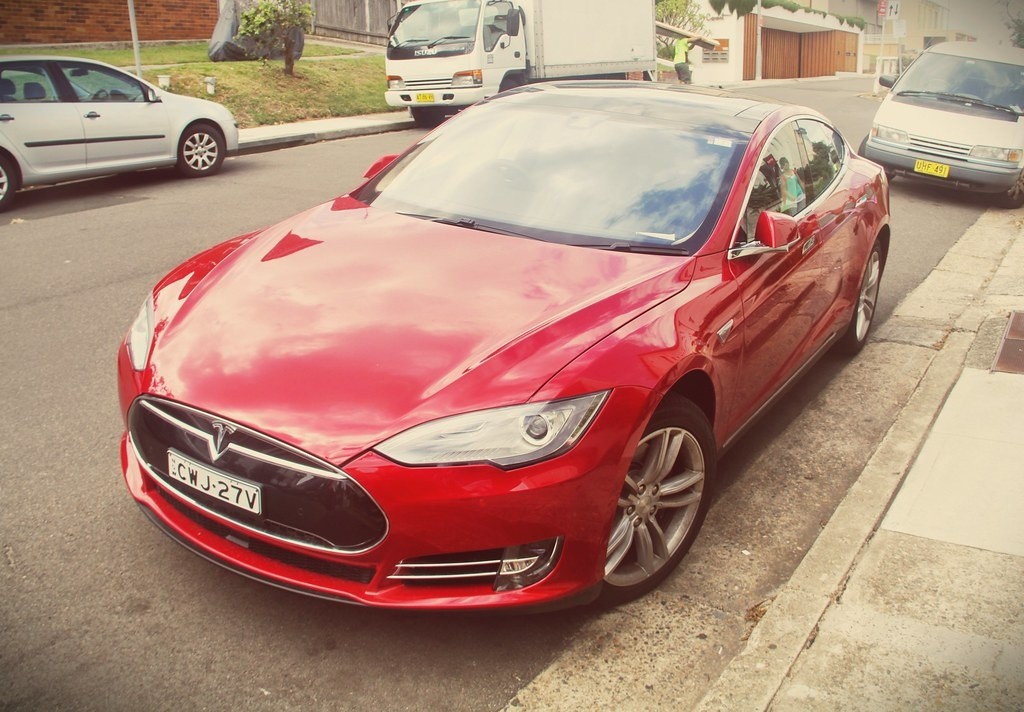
[0,57,239,214]
[116,80,892,615]
[857,45,1023,209]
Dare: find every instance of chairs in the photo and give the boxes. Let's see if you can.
[0,78,17,101]
[24,82,45,99]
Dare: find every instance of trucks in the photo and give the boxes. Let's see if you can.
[384,0,657,125]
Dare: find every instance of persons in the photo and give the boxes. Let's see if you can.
[777,156,805,215]
[674,34,701,84]
